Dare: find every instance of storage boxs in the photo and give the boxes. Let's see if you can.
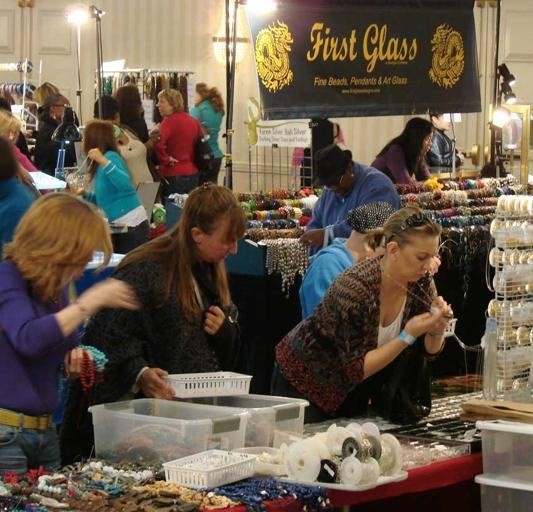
[88,397,249,477]
[171,394,310,448]
[473,474,533,512]
[475,419,533,485]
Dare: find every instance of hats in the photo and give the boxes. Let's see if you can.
[312,143,351,184]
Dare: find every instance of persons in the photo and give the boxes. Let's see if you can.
[367,117,434,185]
[293,144,404,255]
[298,201,398,320]
[58,181,248,469]
[289,116,344,189]
[422,110,465,174]
[1,79,225,256]
[268,205,455,426]
[0,190,145,480]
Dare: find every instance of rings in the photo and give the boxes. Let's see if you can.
[308,239,312,244]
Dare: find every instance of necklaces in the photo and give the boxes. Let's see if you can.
[374,257,440,317]
[77,344,110,392]
[259,237,309,300]
[0,458,330,512]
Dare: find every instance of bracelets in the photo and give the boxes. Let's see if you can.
[398,329,416,347]
[73,299,93,323]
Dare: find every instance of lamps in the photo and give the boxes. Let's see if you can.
[491,62,516,176]
[50,107,83,181]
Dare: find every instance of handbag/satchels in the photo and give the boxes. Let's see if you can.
[195,135,213,169]
[373,352,430,424]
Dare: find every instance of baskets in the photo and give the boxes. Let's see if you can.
[162,449,257,490]
[162,372,253,400]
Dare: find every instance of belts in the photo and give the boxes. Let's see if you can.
[0,409,52,430]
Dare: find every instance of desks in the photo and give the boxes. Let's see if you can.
[0,374,484,512]
